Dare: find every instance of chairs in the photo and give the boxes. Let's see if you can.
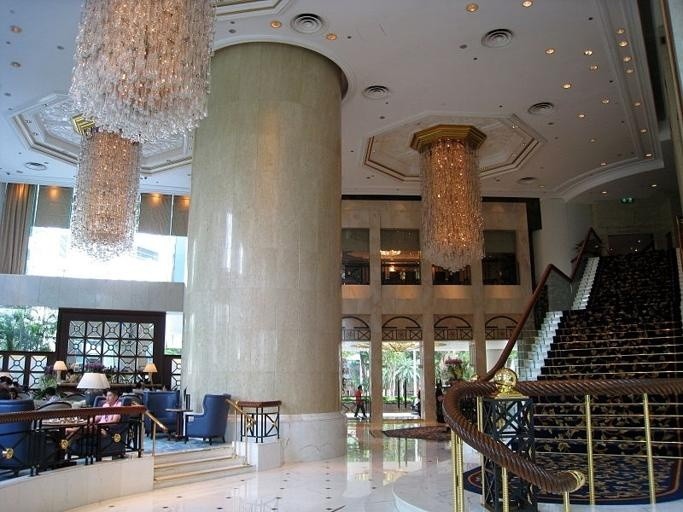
[2,387,234,478]
[388,270,417,284]
[434,271,460,285]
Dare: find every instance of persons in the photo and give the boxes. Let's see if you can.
[40,387,60,407]
[0,376,22,400]
[83,389,122,435]
[354,385,367,418]
[435,383,444,424]
[132,380,151,400]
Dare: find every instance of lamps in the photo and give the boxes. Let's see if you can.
[143,363,158,384]
[409,125,487,273]
[61,1,213,250]
[52,360,68,382]
[77,372,110,405]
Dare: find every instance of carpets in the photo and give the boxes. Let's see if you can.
[458,246,682,508]
[384,423,451,443]
